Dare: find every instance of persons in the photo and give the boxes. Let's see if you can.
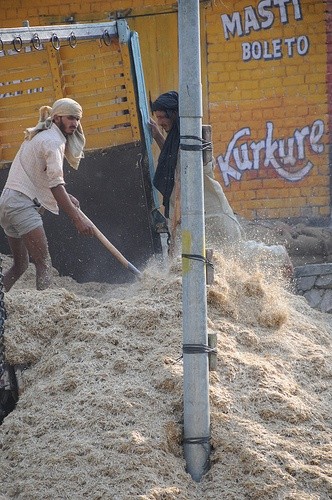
[146,90,206,218]
[0,96,87,294]
